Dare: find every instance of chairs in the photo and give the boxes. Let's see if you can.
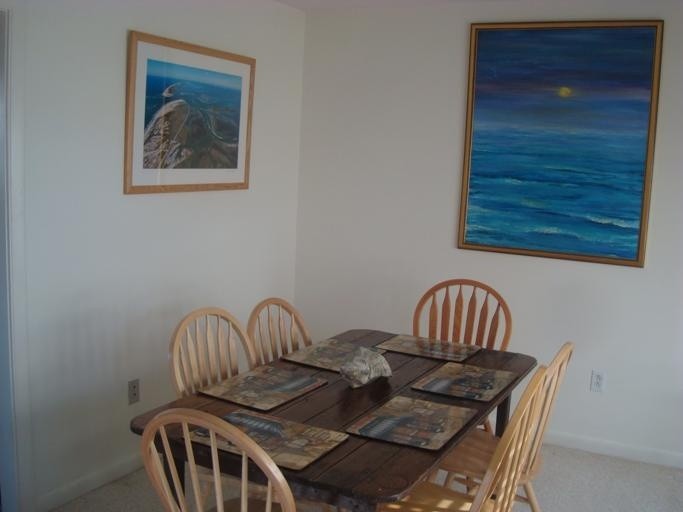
[411,280,513,349]
[142,406,296,512]
[428,340,573,511]
[247,297,311,370]
[375,364,547,512]
[171,306,254,402]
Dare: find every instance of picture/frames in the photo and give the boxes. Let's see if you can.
[122,29,255,196]
[455,19,664,269]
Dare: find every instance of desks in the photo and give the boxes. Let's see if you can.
[130,330,541,510]
